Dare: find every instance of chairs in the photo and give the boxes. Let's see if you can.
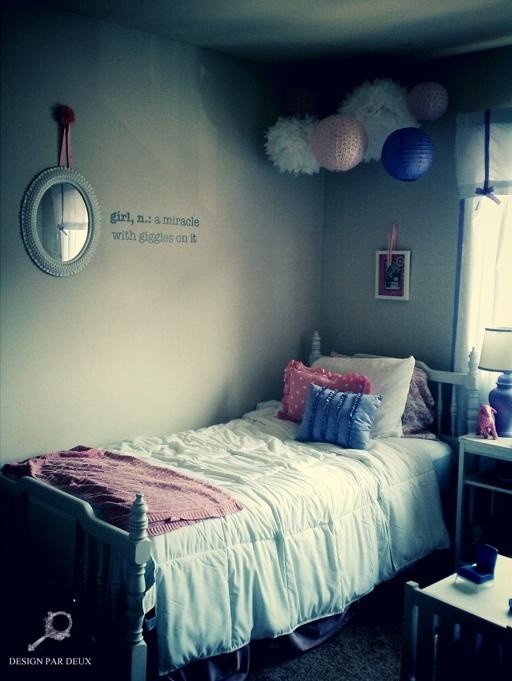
[406,579,512,680]
[453,544,499,590]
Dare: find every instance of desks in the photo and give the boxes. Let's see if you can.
[422,553,512,679]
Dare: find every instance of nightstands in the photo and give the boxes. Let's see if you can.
[452,432,512,568]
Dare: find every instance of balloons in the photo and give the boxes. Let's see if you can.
[310,78,449,183]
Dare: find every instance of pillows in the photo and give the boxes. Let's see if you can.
[309,353,420,441]
[275,356,371,431]
[293,382,384,455]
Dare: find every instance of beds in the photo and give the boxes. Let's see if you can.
[0,329,483,681]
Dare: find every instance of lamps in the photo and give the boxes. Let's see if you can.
[478,327,512,438]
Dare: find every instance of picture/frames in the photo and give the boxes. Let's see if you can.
[374,249,411,304]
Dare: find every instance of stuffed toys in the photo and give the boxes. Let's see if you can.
[476,403,499,440]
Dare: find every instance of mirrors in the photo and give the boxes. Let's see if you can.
[18,166,100,279]
